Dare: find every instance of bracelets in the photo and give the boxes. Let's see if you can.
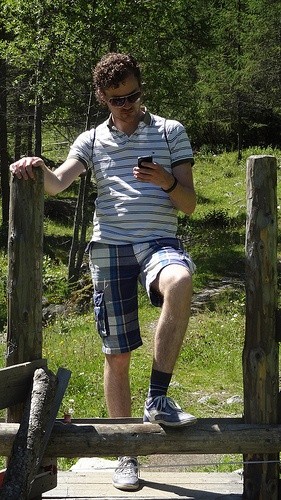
[161,176,178,193]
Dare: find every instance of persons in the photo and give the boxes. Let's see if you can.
[9,52,196,491]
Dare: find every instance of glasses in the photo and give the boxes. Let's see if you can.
[105,86,143,109]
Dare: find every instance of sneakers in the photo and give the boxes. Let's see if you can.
[111,455,141,490]
[142,394,198,427]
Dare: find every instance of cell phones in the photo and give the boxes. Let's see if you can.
[138,155,153,169]
